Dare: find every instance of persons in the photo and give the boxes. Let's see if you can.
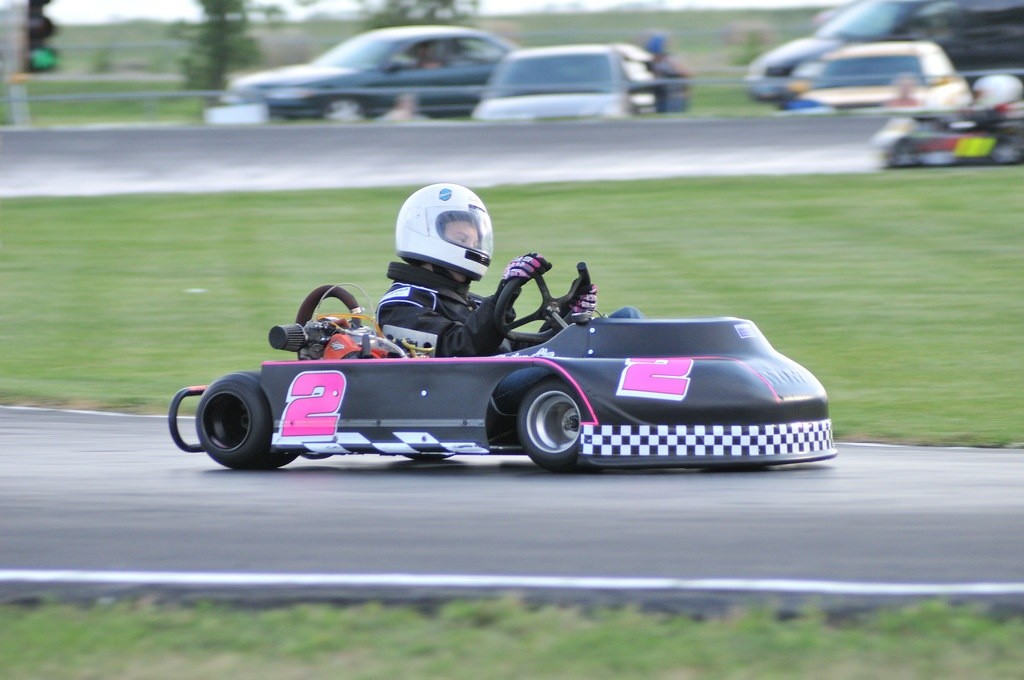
[411,43,442,68]
[376,182,597,357]
[960,75,1024,121]
[646,36,692,113]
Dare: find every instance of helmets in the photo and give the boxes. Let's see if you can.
[970,73,1023,112]
[395,182,493,281]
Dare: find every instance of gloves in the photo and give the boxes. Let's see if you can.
[503,251,549,283]
[569,282,599,325]
